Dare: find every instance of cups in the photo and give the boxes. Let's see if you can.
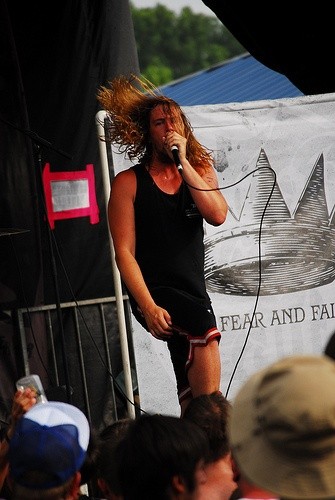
[15,374,48,405]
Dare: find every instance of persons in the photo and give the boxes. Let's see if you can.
[97,76,229,418]
[0,333,335,500]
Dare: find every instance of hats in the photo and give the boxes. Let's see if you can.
[227,355,335,499]
[7,402,91,492]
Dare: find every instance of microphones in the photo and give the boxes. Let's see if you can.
[171,145,183,173]
